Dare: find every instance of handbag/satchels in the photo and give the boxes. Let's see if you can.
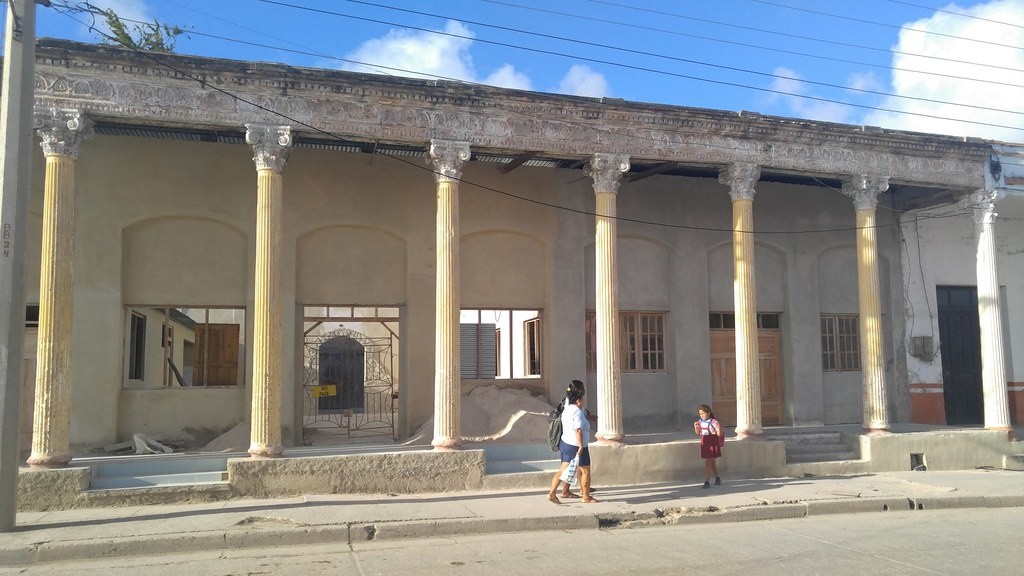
[558,452,580,487]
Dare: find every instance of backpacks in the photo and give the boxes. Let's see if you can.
[712,418,724,447]
[546,406,565,453]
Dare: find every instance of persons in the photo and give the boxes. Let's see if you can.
[560,380,598,496]
[694,404,723,489]
[545,388,597,504]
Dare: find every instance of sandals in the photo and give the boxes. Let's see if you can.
[581,496,599,503]
[580,487,597,495]
[546,492,561,505]
[560,490,580,498]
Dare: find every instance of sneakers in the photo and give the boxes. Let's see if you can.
[702,481,711,489]
[715,478,722,485]
